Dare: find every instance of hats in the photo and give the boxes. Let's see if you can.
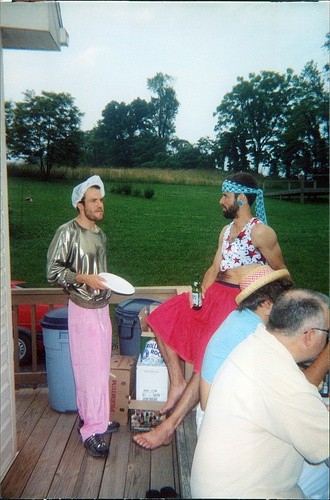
[234,264,291,307]
[71,175,106,209]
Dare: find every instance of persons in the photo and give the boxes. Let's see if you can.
[132,174,286,450]
[191,288,330,500]
[196,264,297,437]
[46,175,120,456]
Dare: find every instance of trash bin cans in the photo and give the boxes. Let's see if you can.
[41,306,78,413]
[114,300,161,356]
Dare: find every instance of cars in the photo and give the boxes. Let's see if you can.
[11,280,68,367]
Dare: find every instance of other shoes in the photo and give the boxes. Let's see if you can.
[160,486,177,499]
[84,433,110,457]
[146,489,162,498]
[79,420,120,435]
